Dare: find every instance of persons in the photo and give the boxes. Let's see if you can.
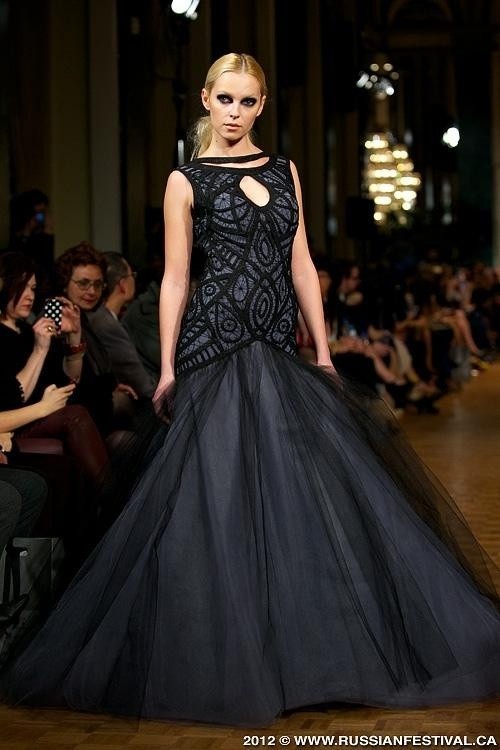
[0,239,500,580]
[0,51,500,728]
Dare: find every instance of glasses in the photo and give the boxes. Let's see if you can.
[70,279,108,292]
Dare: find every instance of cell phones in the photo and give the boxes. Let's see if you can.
[43,297,62,337]
[34,212,45,229]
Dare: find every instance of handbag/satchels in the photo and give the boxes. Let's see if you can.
[0,537,64,605]
